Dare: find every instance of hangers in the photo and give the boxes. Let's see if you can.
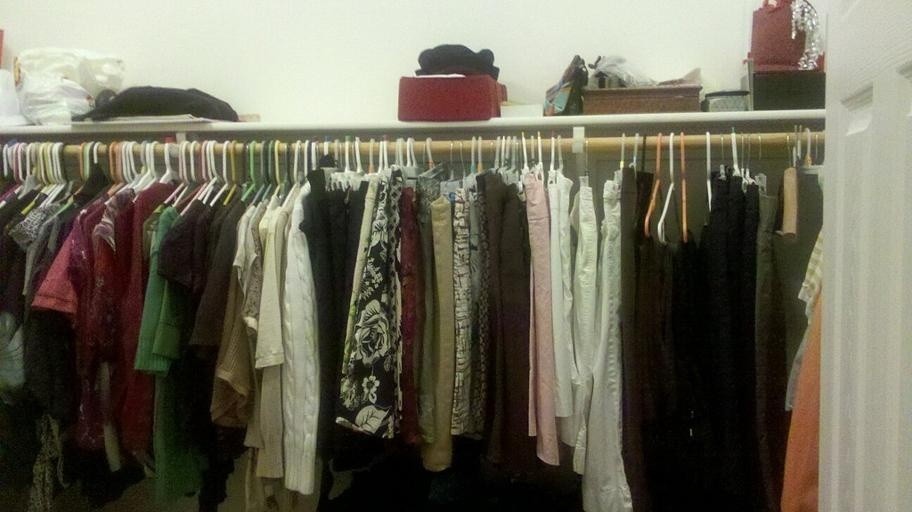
[1,126,826,247]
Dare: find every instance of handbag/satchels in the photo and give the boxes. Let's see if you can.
[751,0,824,71]
[543,54,587,116]
[71,87,238,124]
